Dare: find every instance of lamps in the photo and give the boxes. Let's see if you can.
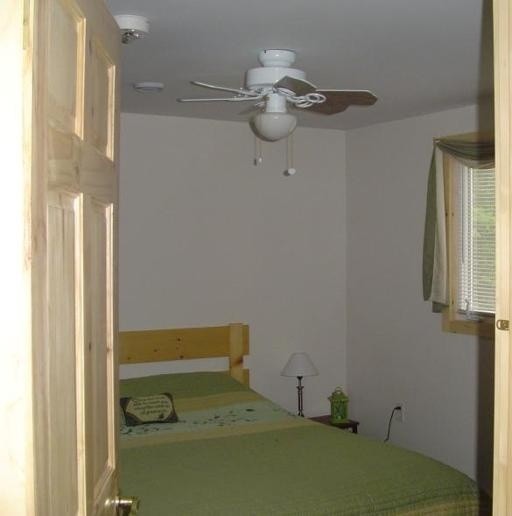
[279,351,319,417]
[248,94,300,141]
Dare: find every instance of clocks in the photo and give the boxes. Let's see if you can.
[327,385,350,425]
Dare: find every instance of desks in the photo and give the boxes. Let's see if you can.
[307,414,360,434]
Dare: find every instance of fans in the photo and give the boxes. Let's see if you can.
[172,47,379,116]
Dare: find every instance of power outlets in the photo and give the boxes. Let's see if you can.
[391,404,405,422]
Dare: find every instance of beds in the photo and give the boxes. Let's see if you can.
[118,323,491,515]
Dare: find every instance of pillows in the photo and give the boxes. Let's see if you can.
[119,391,178,428]
